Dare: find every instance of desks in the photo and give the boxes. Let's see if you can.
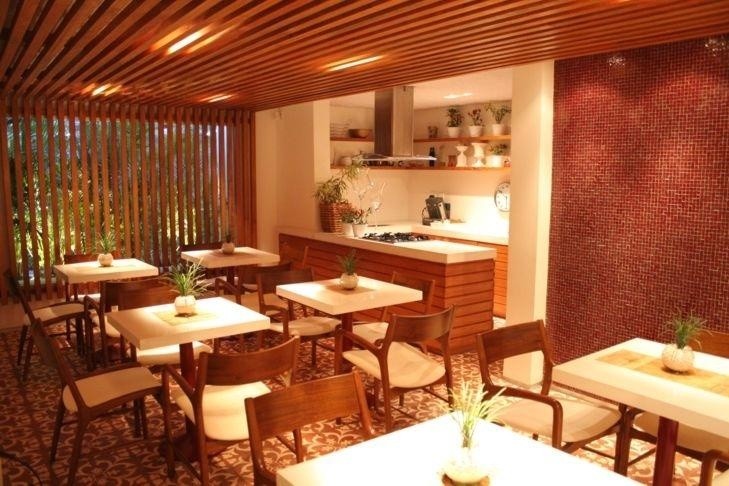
[552,338,728,486]
[275,408,643,486]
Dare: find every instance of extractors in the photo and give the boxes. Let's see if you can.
[351,85,438,162]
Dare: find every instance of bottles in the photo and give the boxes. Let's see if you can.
[429,148,435,166]
[447,155,457,167]
[428,126,438,138]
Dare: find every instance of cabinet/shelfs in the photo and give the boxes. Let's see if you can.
[330,135,512,171]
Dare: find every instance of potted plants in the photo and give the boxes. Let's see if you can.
[96,231,115,266]
[445,102,462,137]
[485,101,511,135]
[313,165,372,237]
[657,307,712,373]
[490,144,507,167]
[438,379,521,485]
[467,108,484,137]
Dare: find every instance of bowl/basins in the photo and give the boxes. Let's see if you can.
[350,128,372,138]
[340,157,353,166]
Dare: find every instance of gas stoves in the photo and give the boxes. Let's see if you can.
[361,231,430,243]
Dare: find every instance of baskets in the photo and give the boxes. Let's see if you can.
[320,198,352,234]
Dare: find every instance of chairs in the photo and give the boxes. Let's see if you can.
[620,326,729,486]
[475,319,628,473]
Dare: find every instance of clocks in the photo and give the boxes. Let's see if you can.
[494,182,511,212]
